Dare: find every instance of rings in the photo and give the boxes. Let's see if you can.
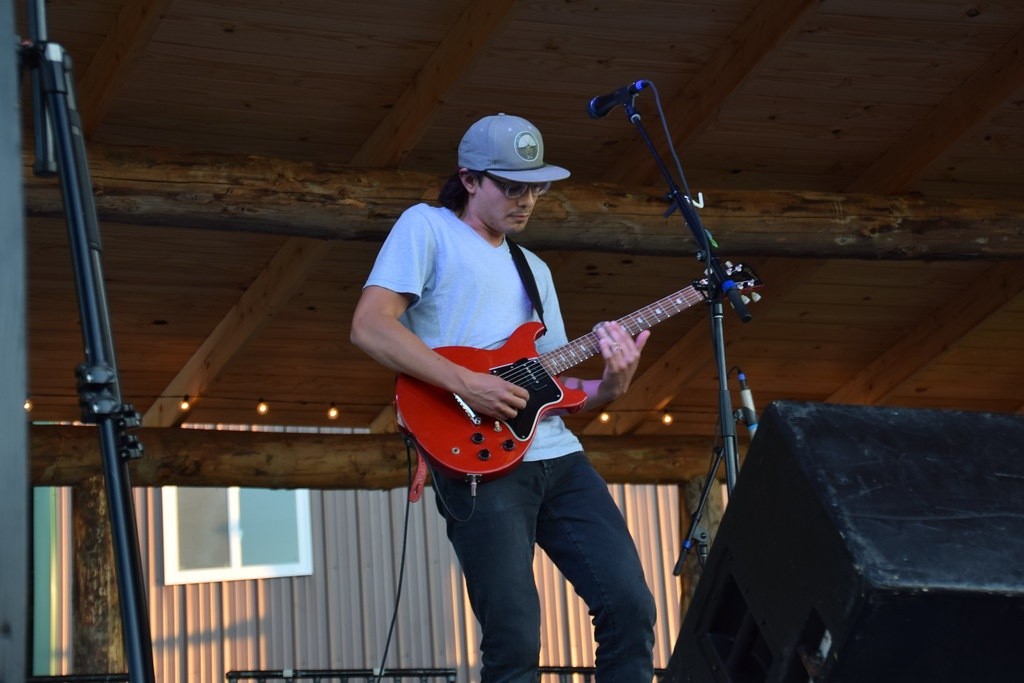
[610,342,622,352]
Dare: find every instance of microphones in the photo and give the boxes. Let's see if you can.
[586,79,650,119]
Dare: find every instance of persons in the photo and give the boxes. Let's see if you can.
[348,112,658,682]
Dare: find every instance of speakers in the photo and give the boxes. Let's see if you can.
[659,399,1024,681]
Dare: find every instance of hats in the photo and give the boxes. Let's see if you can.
[458,113,572,183]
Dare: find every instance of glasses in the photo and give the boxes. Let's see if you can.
[483,172,552,199]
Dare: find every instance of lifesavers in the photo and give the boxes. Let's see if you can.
[392,258,768,490]
